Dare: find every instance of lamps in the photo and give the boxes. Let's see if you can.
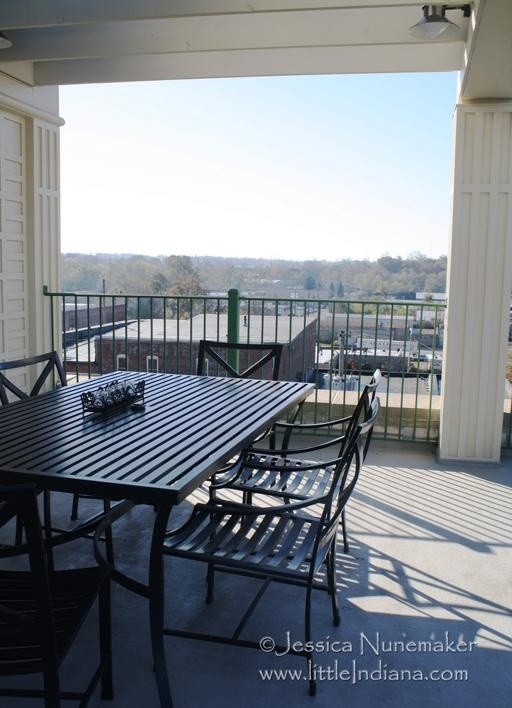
[405,4,472,42]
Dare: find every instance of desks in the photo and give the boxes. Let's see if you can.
[1,368,319,707]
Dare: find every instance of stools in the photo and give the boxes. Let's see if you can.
[1,480,116,706]
[2,479,54,571]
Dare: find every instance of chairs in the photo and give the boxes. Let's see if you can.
[204,367,383,597]
[1,350,116,571]
[160,397,381,697]
[195,338,284,492]
